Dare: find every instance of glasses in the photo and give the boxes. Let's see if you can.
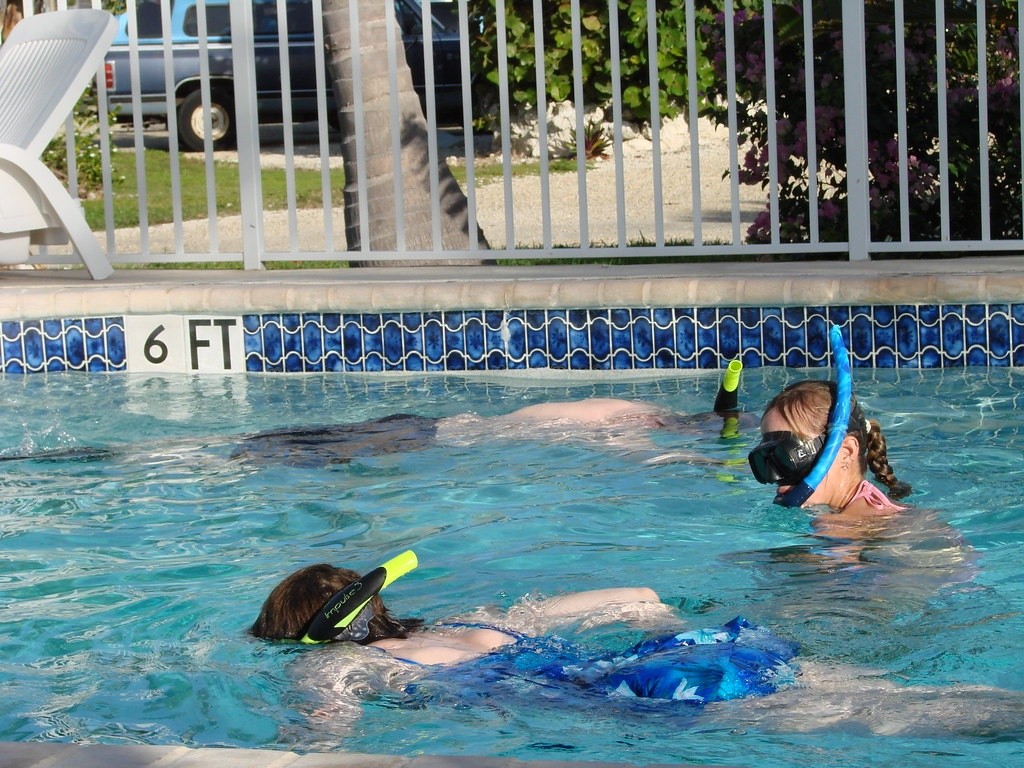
[748,403,861,485]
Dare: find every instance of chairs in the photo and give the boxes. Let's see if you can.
[0,9,118,280]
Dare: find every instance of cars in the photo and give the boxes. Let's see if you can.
[105,0,495,153]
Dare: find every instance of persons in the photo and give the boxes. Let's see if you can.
[248,560,1024,751]
[231,396,765,485]
[758,379,916,519]
